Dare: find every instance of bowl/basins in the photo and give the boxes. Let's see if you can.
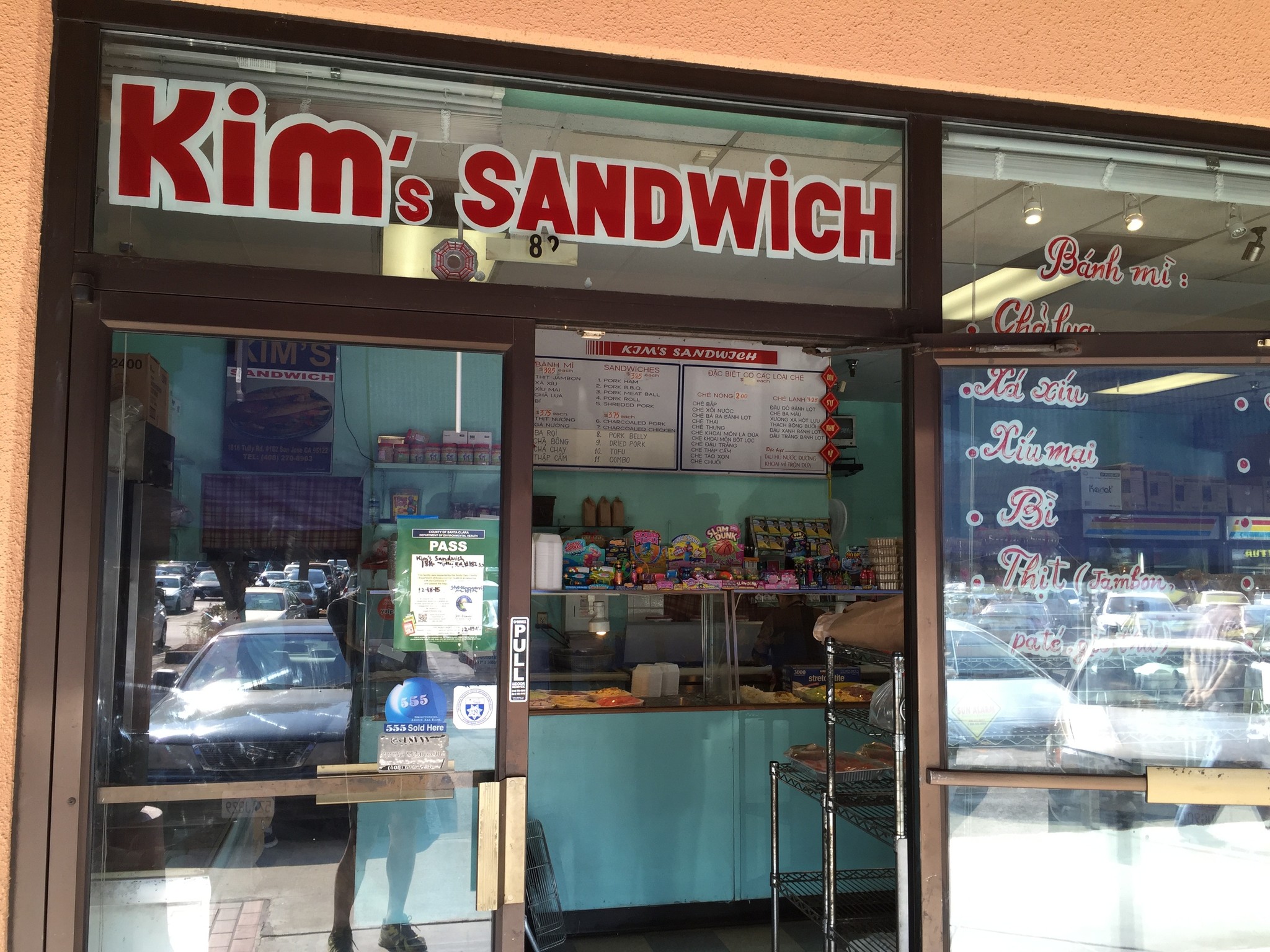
[531,532,563,589]
[631,662,680,698]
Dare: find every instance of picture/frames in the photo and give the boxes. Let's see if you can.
[378,435,405,445]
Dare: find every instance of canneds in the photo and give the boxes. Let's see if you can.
[449,502,500,519]
[376,430,502,466]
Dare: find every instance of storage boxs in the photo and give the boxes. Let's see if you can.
[781,664,862,694]
[443,429,468,444]
[1030,462,1270,514]
[110,352,170,433]
[468,432,492,464]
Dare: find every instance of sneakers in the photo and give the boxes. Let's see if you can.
[327,927,359,952]
[378,912,427,952]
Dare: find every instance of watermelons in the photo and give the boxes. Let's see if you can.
[802,755,874,773]
[877,757,906,769]
[597,695,640,706]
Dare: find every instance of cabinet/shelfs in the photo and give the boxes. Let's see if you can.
[770,638,909,952]
[374,460,501,532]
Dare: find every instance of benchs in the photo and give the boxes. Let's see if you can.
[269,651,333,684]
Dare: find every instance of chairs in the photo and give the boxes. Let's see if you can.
[332,653,346,676]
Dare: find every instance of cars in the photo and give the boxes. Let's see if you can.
[945,580,1270,817]
[148,620,351,835]
[154,558,358,619]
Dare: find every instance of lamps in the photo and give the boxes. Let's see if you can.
[1123,193,1145,231]
[587,595,611,637]
[1020,184,1045,226]
[1224,203,1247,238]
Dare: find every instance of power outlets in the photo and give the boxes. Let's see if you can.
[537,613,549,631]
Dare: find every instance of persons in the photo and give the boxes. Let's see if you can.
[326,527,433,952]
[751,593,828,694]
